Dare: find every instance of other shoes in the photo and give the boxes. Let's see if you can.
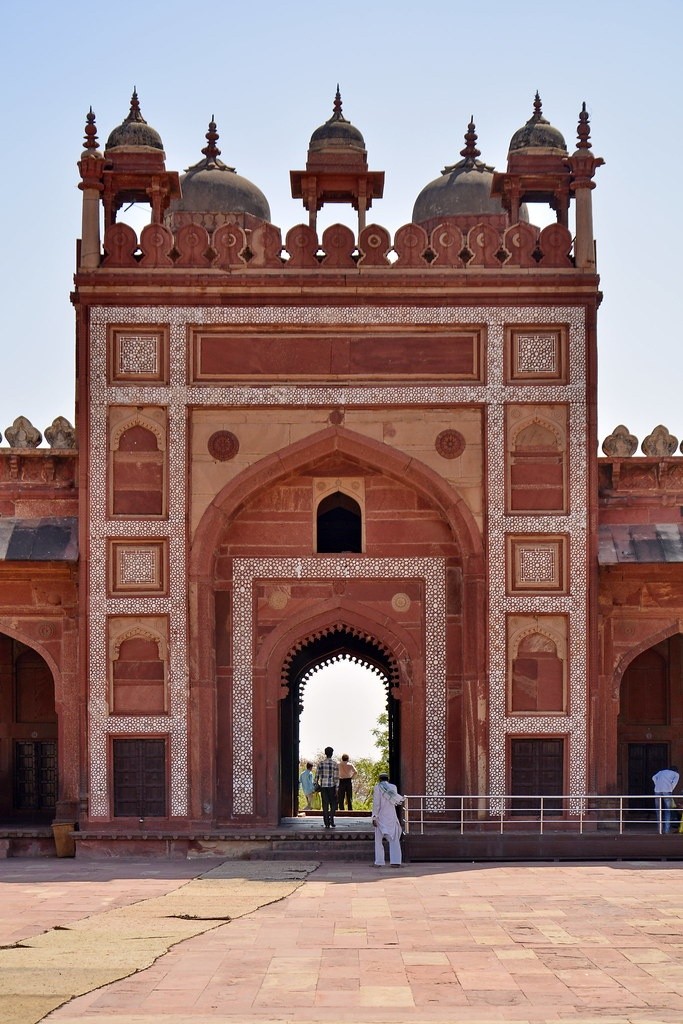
[329,820,336,827]
[325,824,330,828]
[369,864,382,868]
[392,864,404,868]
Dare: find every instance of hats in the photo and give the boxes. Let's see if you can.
[379,773,388,779]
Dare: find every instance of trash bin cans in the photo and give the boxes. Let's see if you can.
[51,823,75,857]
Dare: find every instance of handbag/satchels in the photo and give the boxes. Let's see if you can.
[383,788,403,805]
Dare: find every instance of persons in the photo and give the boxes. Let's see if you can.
[368,772,402,868]
[652,765,679,833]
[338,754,357,810]
[314,747,339,828]
[300,763,315,811]
